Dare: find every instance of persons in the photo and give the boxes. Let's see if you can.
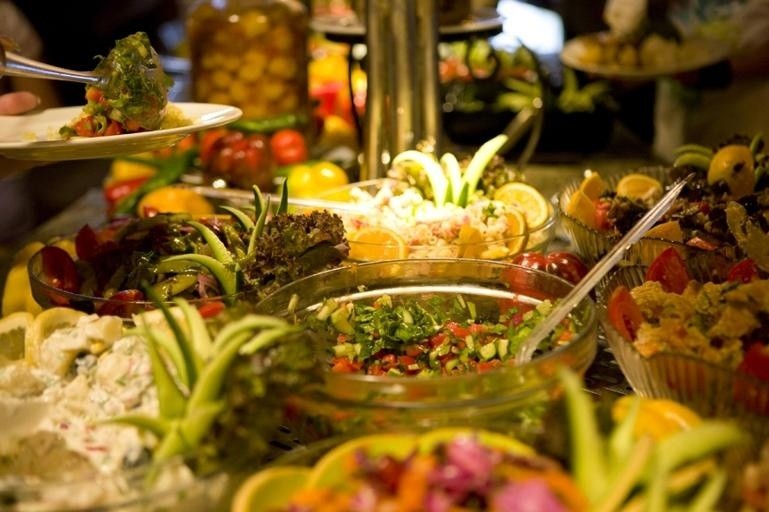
[0,1,63,239]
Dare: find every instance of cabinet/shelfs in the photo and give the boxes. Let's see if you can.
[186,0,315,154]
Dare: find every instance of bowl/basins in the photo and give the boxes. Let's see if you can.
[555,172,769,271]
[242,431,754,512]
[311,172,560,266]
[11,325,227,510]
[30,187,311,315]
[257,266,604,440]
[606,257,769,426]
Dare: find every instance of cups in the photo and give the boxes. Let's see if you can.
[347,1,441,173]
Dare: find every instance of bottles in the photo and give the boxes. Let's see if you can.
[186,0,315,154]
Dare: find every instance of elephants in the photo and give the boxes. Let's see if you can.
[30,246,79,305]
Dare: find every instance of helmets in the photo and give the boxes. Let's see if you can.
[0,101,243,163]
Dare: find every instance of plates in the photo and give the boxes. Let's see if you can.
[0,101,243,163]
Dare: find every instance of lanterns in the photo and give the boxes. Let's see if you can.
[0,307,83,366]
[505,207,529,256]
[494,181,549,228]
[351,228,409,279]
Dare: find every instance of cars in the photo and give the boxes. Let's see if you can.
[347,1,441,173]
